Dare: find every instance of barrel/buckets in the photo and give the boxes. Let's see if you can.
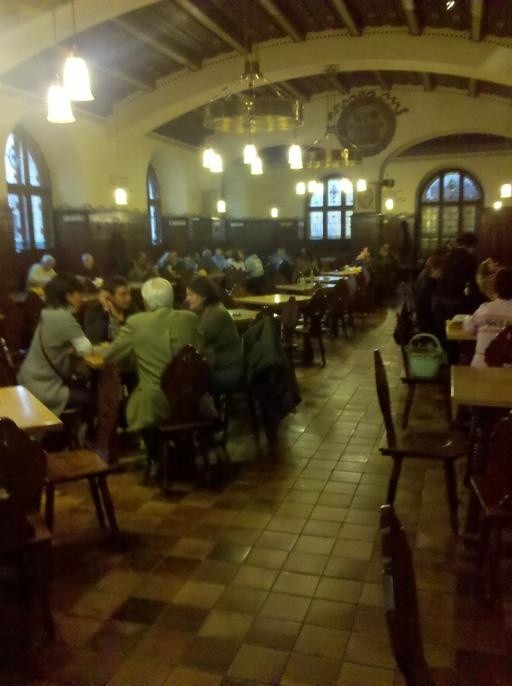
[403,333,445,381]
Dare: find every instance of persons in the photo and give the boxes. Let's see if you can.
[13,271,103,454]
[184,276,241,360]
[352,230,512,367]
[102,277,202,482]
[74,252,117,292]
[129,246,320,308]
[81,273,143,344]
[21,250,61,292]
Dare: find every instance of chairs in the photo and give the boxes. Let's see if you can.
[371,500,510,685]
[139,344,232,499]
[46,360,122,531]
[1,415,63,645]
[463,405,511,594]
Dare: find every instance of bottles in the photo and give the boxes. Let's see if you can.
[296,270,315,288]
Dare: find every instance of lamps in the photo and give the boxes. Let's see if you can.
[199,1,306,178]
[44,0,97,128]
[294,75,367,195]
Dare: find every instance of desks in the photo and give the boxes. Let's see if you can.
[1,386,63,447]
[83,356,140,472]
[32,268,225,311]
[225,267,370,368]
